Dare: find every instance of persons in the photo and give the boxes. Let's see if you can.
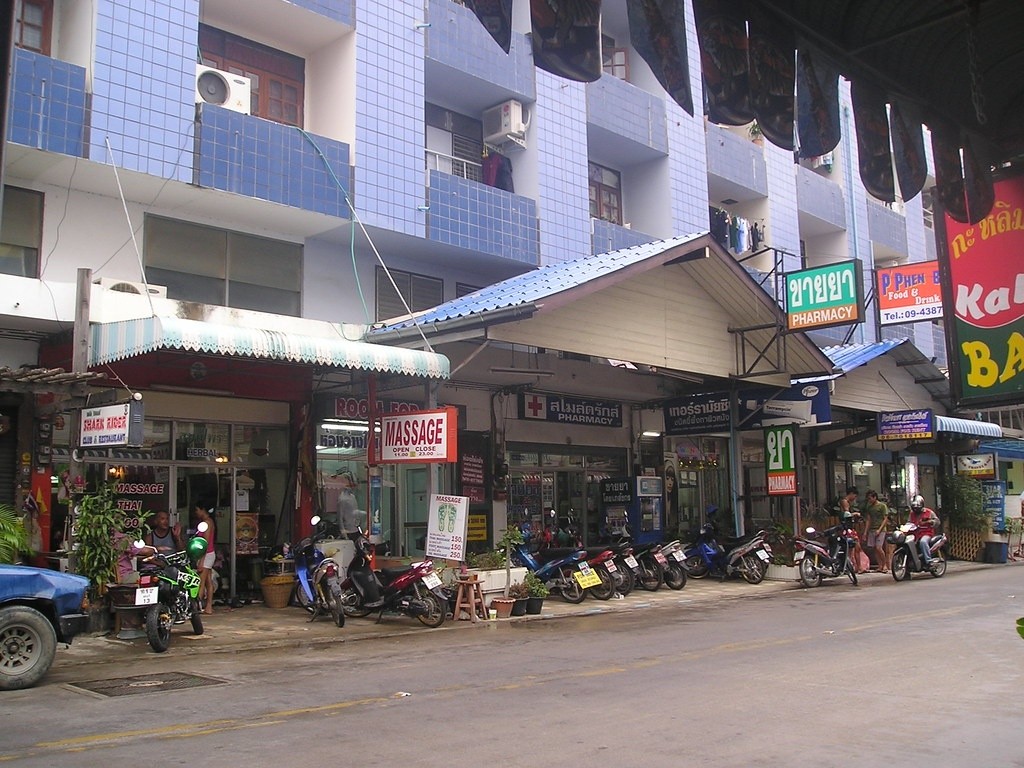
[144,510,187,623]
[907,494,940,572]
[108,518,154,584]
[337,487,360,543]
[193,501,217,615]
[862,488,890,574]
[665,459,679,528]
[841,486,867,575]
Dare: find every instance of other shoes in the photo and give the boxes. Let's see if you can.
[928,561,934,567]
[874,569,888,574]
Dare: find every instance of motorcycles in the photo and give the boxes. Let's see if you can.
[789,511,858,588]
[537,509,690,600]
[498,508,602,605]
[886,514,948,583]
[133,521,209,653]
[340,525,449,628]
[285,515,345,627]
[684,507,774,585]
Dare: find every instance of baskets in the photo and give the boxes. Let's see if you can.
[260,576,295,607]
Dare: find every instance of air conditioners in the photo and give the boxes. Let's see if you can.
[876,260,898,267]
[95,278,167,298]
[194,61,252,116]
[480,98,525,144]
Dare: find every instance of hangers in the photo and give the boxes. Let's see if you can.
[716,206,764,228]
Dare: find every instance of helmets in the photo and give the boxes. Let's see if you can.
[893,531,906,544]
[911,495,925,507]
[187,537,209,560]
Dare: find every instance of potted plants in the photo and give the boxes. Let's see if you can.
[746,122,762,146]
[73,496,124,633]
[462,524,546,619]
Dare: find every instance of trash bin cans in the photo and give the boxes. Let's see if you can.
[985,540,1009,563]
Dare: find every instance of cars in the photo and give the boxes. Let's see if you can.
[0,564,92,691]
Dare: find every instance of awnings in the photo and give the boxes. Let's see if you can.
[931,413,1003,444]
[86,315,450,397]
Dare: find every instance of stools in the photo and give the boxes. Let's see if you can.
[453,580,488,623]
